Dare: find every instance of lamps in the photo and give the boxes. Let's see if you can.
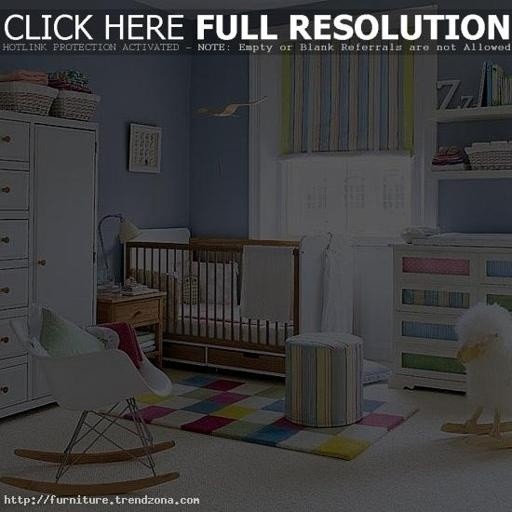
[98,214,143,292]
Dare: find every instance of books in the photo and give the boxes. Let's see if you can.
[475,61,512,108]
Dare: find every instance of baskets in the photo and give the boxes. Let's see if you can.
[467,149,512,171]
[51,91,101,121]
[0,83,58,116]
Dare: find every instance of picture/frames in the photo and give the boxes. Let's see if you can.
[129,122,162,173]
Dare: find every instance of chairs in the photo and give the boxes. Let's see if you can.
[0,320,180,497]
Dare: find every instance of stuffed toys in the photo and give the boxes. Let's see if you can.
[441,305,512,450]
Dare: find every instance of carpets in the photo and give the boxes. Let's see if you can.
[104,367,420,462]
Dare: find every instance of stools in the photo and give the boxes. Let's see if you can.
[285,331,365,427]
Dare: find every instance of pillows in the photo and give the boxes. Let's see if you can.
[185,260,238,307]
[363,359,393,383]
[40,306,106,356]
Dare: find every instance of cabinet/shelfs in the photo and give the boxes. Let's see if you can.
[417,53,512,234]
[97,293,166,369]
[0,109,99,419]
[388,239,512,392]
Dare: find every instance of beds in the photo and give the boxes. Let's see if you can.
[124,228,354,377]
